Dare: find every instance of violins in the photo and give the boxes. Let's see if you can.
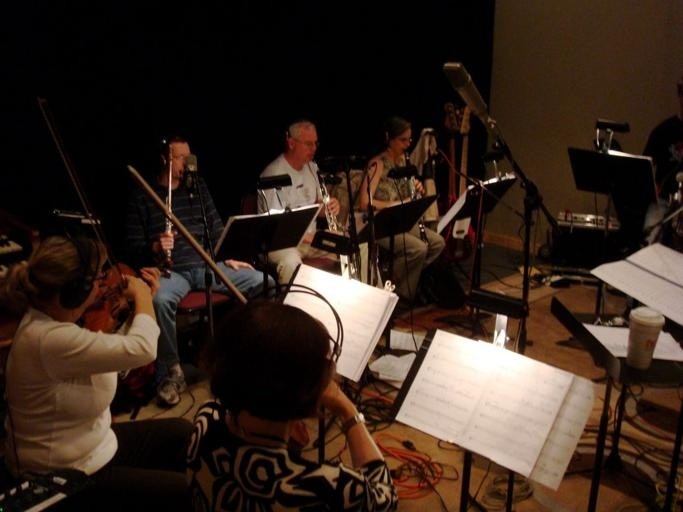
[80,261,150,334]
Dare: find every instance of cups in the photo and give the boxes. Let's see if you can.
[628,308,666,369]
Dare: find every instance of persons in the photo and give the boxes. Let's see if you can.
[258,119,340,244]
[643,76,683,199]
[359,115,446,306]
[127,136,276,405]
[187,300,397,512]
[1,234,193,511]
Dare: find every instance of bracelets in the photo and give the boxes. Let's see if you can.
[340,411,366,434]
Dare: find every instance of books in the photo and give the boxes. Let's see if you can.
[589,240,683,328]
[395,328,597,492]
[282,265,399,384]
[437,172,517,237]
[214,203,320,259]
[569,146,659,200]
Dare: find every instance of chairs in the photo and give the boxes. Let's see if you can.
[174,290,232,354]
[330,168,365,226]
[235,193,344,279]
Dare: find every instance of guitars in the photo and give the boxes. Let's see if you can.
[437,104,457,216]
[444,106,476,263]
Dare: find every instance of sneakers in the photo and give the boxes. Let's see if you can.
[158,370,188,404]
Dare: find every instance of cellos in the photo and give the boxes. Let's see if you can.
[660,169,683,254]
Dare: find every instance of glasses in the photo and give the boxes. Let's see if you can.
[291,137,320,148]
[96,272,108,280]
[393,136,413,143]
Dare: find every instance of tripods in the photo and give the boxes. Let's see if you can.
[448,211,534,346]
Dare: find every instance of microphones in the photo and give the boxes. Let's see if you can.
[676,172,683,207]
[184,155,198,199]
[443,61,490,128]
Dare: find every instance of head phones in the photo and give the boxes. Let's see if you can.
[382,130,390,144]
[58,237,101,310]
[152,135,174,173]
[202,282,344,394]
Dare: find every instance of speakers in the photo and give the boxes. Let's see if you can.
[552,222,621,270]
